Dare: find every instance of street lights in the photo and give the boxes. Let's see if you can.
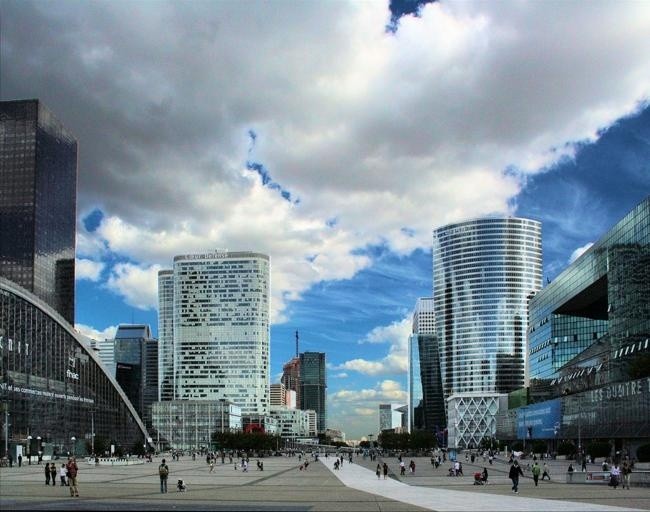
[27,435,33,464]
[36,435,42,464]
[1,394,13,455]
[142,413,148,453]
[88,407,97,456]
[71,436,77,456]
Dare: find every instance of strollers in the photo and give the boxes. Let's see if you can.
[474,471,485,485]
[449,467,456,477]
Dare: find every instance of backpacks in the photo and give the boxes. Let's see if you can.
[158,464,168,478]
